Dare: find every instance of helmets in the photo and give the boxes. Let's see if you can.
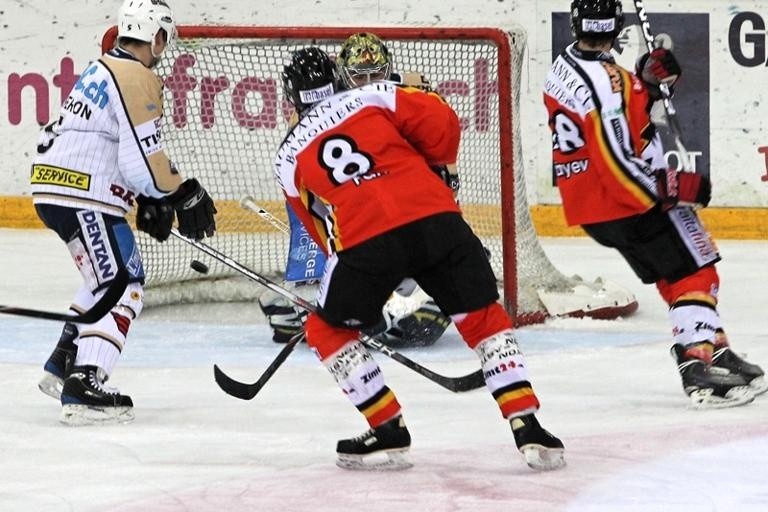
[282,47,336,119]
[569,0,625,48]
[116,0,176,58]
[335,32,393,90]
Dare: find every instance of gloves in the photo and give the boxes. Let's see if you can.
[662,165,714,211]
[634,47,683,102]
[171,176,218,244]
[135,193,176,245]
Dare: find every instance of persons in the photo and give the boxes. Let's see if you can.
[276,49,567,470]
[31,2,217,425]
[292,31,460,344]
[544,1,768,410]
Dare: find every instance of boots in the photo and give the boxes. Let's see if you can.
[507,412,567,474]
[57,368,136,429]
[707,344,768,398]
[671,349,757,412]
[36,322,77,403]
[334,414,415,471]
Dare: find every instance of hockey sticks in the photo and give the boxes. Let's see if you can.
[213,332,306,400]
[240,194,432,319]
[0,266,130,323]
[143,211,486,393]
